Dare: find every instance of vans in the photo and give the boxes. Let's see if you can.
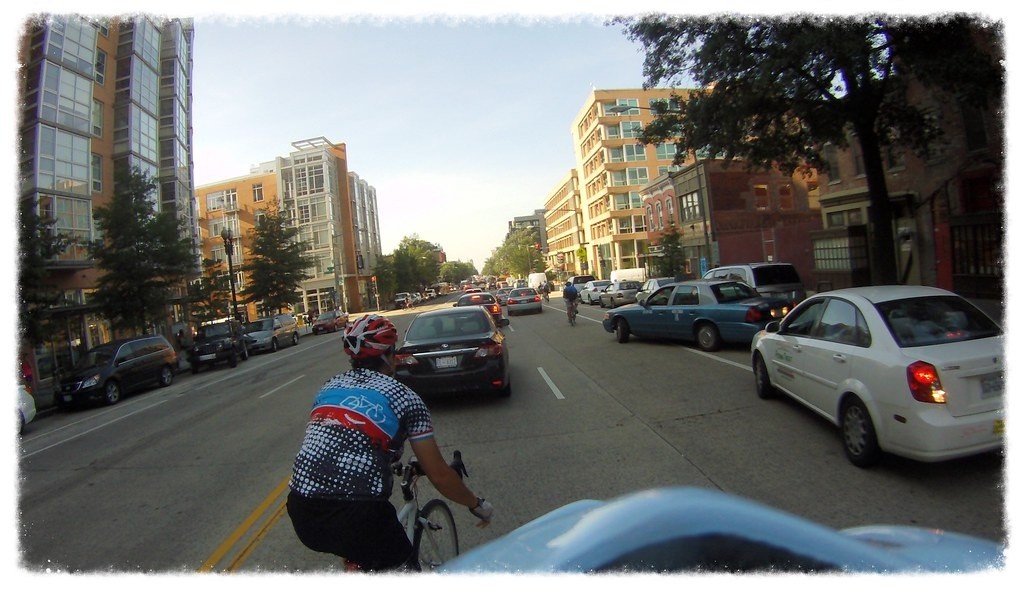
[54,334,176,410]
[565,274,595,297]
[702,262,806,302]
[244,314,300,354]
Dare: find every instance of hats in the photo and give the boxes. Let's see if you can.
[564,282,571,285]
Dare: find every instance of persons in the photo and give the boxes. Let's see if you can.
[404,296,411,309]
[176,329,190,361]
[563,282,579,323]
[537,280,549,302]
[286,314,494,573]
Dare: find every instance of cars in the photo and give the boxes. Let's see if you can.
[19,383,36,437]
[579,279,1005,467]
[453,280,502,318]
[488,281,542,316]
[393,306,510,401]
[413,288,436,305]
[312,310,349,335]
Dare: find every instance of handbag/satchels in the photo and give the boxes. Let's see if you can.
[563,289,577,301]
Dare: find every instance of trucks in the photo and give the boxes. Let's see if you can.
[394,292,412,309]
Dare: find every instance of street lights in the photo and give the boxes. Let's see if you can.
[606,103,712,269]
[525,209,587,275]
[332,228,367,309]
[221,227,241,319]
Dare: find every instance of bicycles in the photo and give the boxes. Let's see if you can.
[343,449,468,575]
[543,289,550,303]
[567,300,576,325]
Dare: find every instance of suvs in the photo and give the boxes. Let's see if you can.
[188,317,248,373]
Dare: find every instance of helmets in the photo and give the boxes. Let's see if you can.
[341,313,398,359]
